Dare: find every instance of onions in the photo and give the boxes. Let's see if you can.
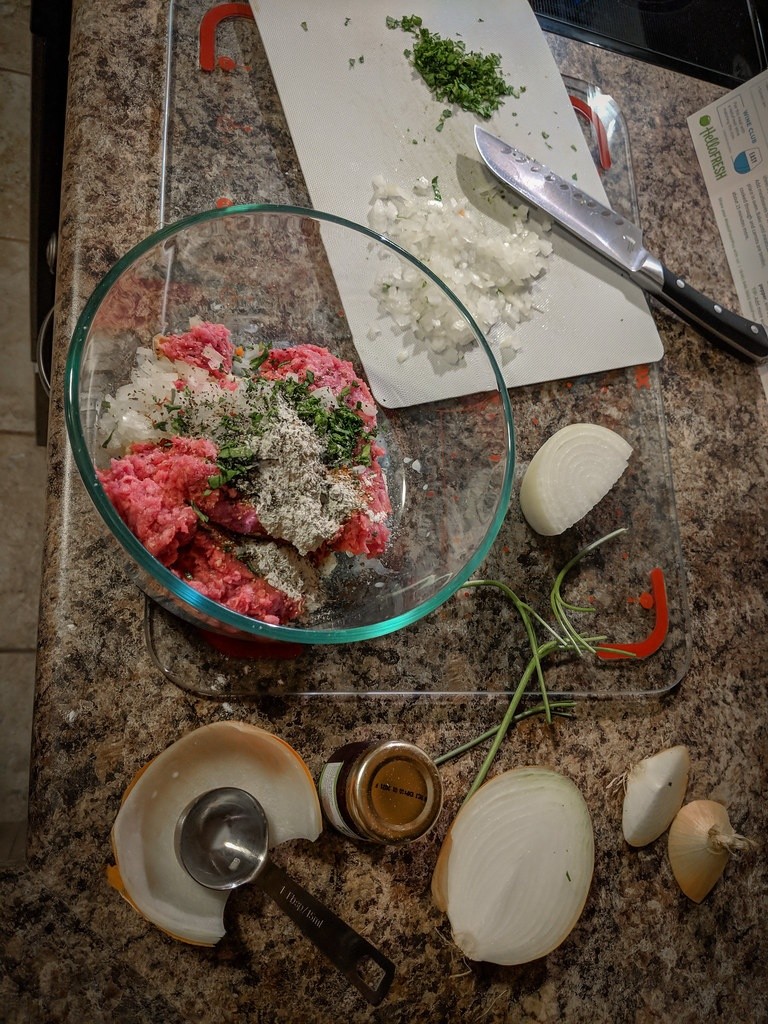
[519,422,634,536]
[106,718,751,965]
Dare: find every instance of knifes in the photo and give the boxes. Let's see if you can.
[473,125,768,368]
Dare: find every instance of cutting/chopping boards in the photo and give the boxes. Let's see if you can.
[250,1,665,410]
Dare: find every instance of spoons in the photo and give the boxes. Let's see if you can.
[174,786,394,1006]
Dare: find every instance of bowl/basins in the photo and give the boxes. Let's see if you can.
[62,203,518,640]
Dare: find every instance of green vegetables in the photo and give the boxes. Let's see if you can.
[433,525,628,805]
